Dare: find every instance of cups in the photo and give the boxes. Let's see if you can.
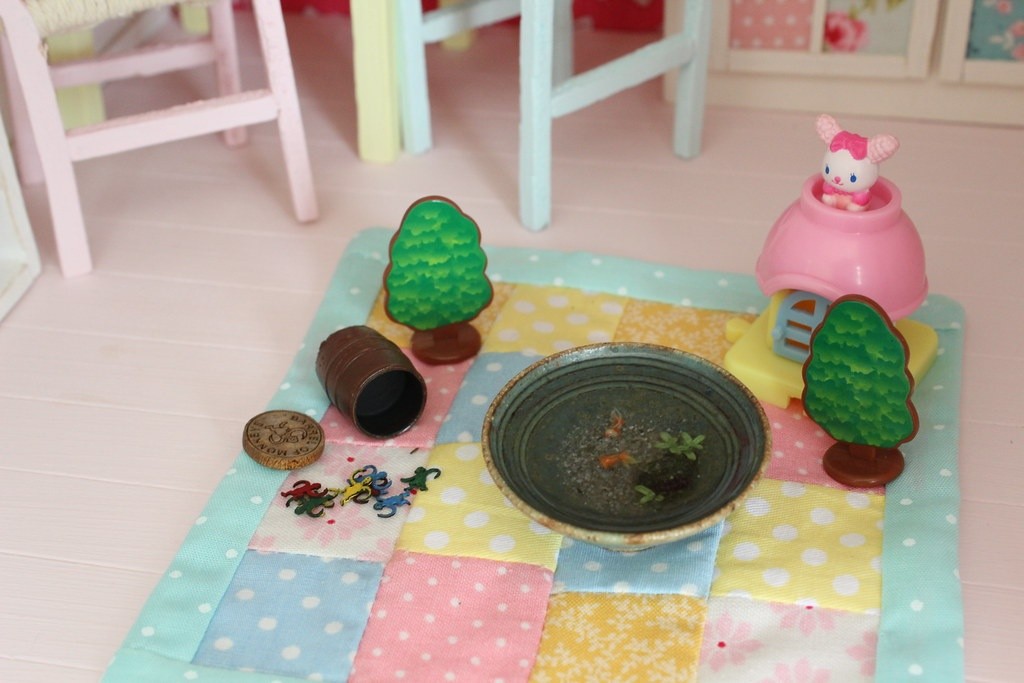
[316,326,425,439]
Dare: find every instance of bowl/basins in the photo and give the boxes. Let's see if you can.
[481,342,772,549]
